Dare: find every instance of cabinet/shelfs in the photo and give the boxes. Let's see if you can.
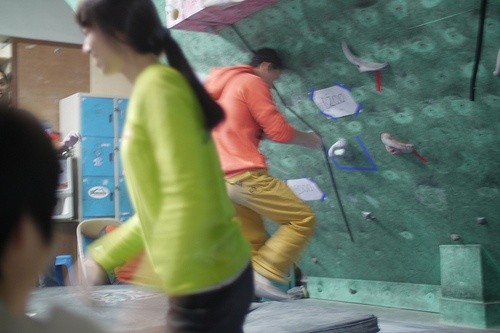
[59,92,134,223]
[52,157,75,222]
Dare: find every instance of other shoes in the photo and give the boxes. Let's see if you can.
[253,279,287,300]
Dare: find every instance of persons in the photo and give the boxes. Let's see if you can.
[203,47,322,301]
[70,0,254,333]
[0,103,100,333]
